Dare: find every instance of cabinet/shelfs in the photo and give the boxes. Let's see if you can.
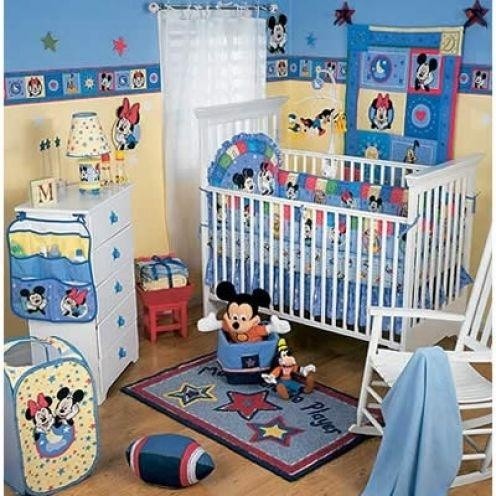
[14,182,140,410]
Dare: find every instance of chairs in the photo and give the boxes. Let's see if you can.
[347,220,494,489]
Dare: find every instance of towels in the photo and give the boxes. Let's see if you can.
[135,257,188,292]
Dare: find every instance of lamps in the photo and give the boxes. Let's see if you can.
[65,111,112,195]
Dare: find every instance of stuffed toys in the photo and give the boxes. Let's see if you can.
[261,338,316,399]
[197,281,291,344]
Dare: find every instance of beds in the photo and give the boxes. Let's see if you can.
[192,95,487,377]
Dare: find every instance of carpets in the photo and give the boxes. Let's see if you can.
[118,351,386,483]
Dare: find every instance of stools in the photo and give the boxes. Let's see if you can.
[135,294,195,344]
